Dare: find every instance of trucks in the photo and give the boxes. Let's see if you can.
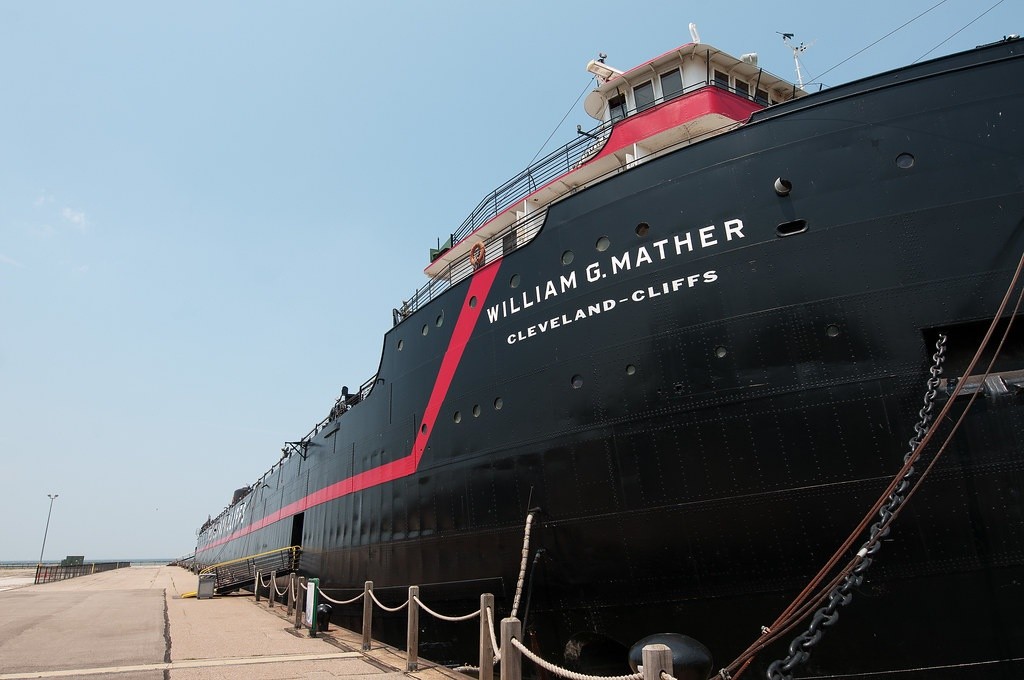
[61,556,84,566]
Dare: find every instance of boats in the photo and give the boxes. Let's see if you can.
[194,20,1024,680]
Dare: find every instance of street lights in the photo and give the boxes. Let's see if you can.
[40,494,59,563]
